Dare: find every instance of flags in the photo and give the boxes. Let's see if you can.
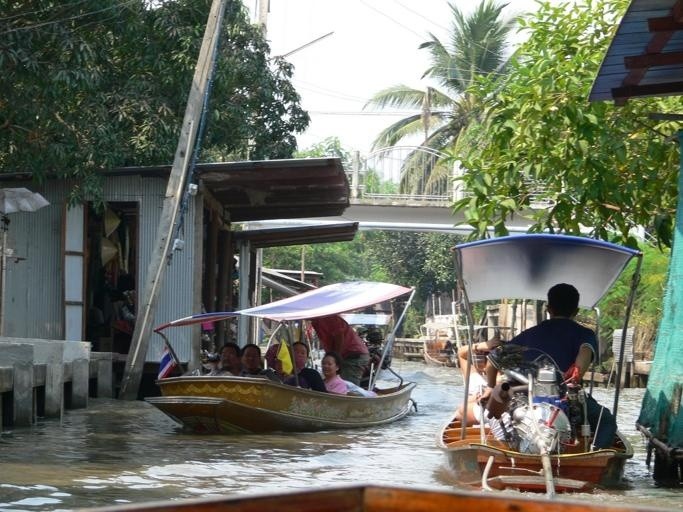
[156,341,177,379]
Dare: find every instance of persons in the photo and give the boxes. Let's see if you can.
[238,344,283,383]
[306,314,370,386]
[322,349,349,394]
[458,337,516,426]
[290,341,328,392]
[445,341,453,355]
[452,342,457,353]
[477,283,616,449]
[206,342,242,376]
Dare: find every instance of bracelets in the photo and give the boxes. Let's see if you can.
[486,383,495,387]
